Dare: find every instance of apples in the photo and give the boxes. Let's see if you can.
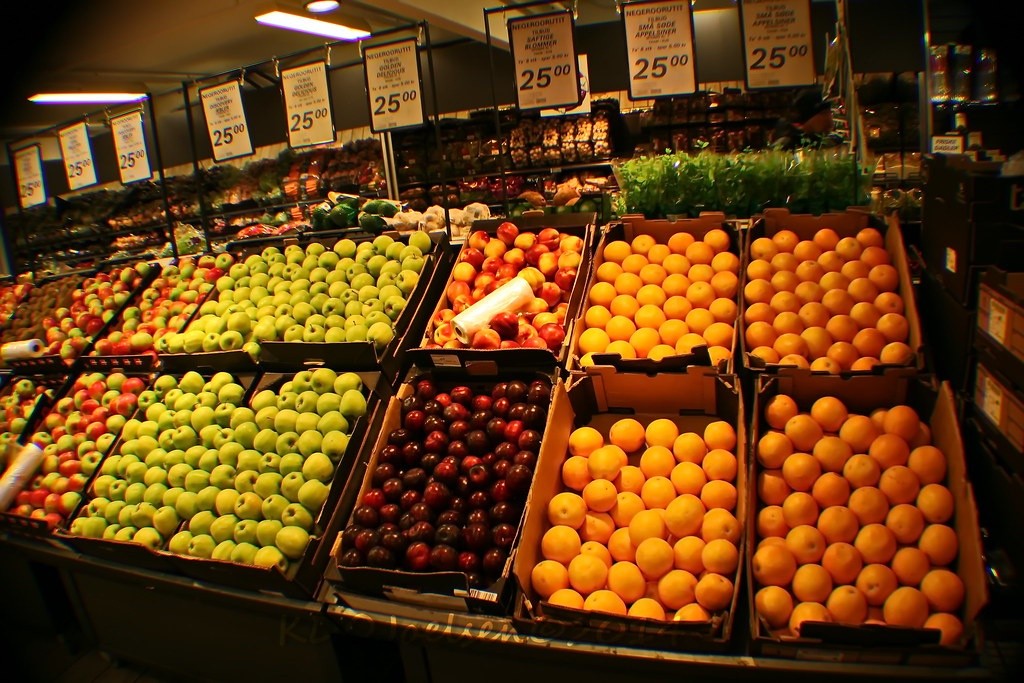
[0,230,432,572]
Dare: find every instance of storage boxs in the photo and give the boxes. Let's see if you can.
[1,197,992,681]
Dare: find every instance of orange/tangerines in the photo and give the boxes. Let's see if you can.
[532,229,963,647]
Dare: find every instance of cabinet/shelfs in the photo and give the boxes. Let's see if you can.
[1,38,1023,281]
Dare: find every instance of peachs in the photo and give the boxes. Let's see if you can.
[431,221,583,357]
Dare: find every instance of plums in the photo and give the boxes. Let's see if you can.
[340,378,550,588]
